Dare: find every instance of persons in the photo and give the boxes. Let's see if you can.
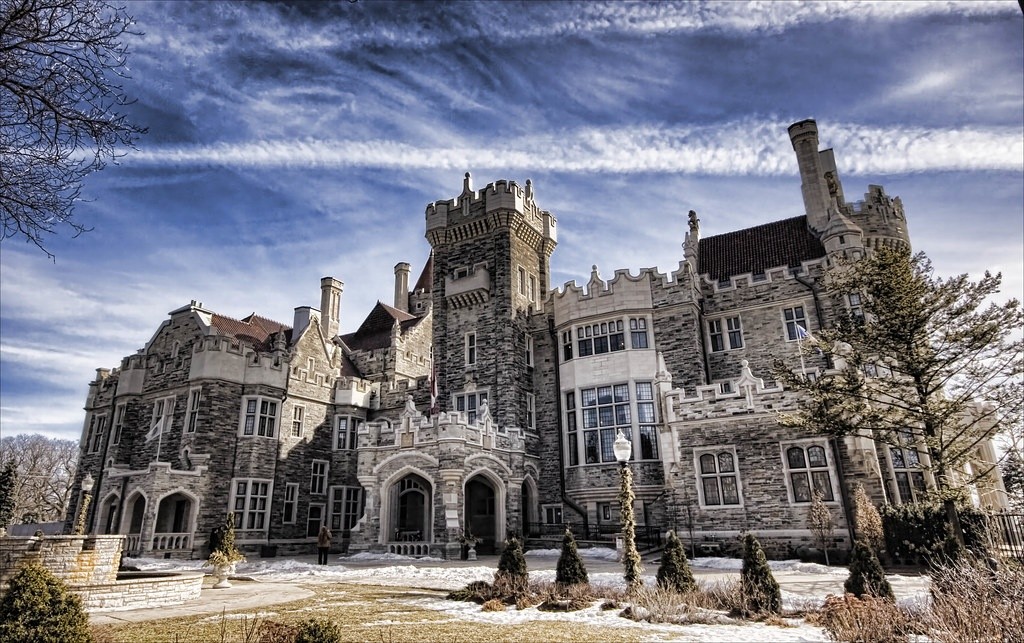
[318,526,332,565]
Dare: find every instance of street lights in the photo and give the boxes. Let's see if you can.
[613,428,642,589]
[73,472,95,536]
